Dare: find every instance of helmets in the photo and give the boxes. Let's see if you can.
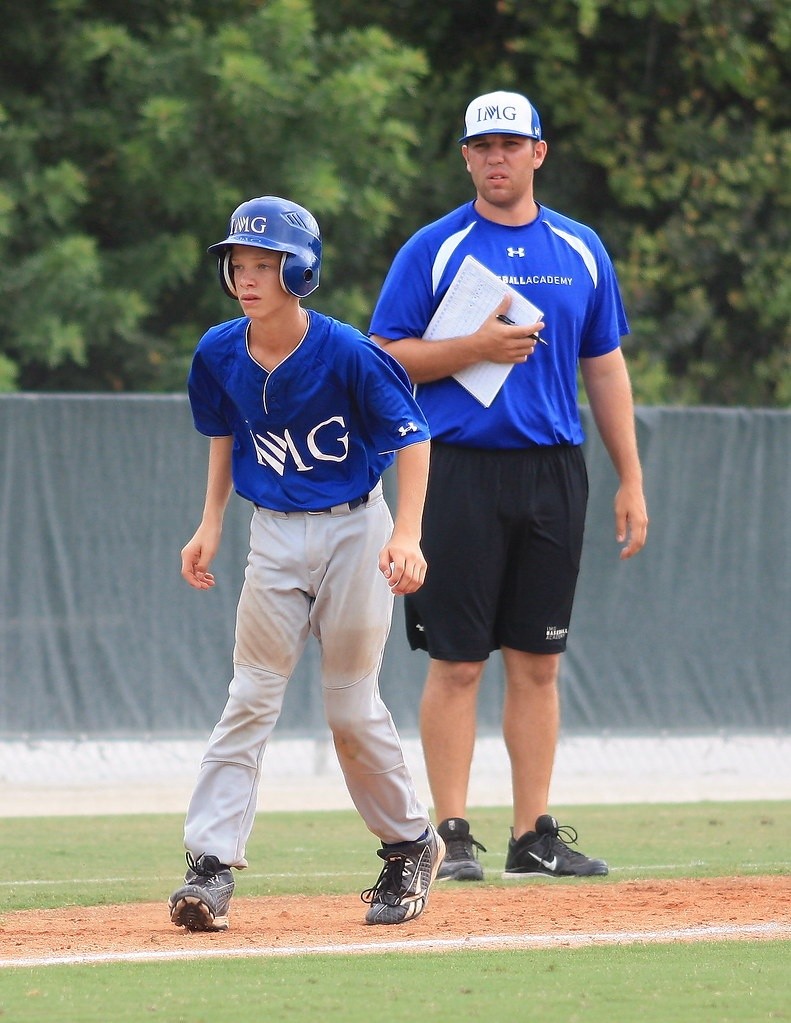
[207,193,323,300]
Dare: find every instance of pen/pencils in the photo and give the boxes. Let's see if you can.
[495,314,550,348]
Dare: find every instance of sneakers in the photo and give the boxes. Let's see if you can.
[502,814,609,880]
[360,823,446,926]
[168,851,235,931]
[436,817,488,881]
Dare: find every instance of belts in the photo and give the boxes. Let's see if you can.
[308,494,369,516]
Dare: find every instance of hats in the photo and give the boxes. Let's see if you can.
[460,90,542,144]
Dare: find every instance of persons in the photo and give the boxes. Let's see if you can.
[369,90,649,881]
[168,196,446,932]
